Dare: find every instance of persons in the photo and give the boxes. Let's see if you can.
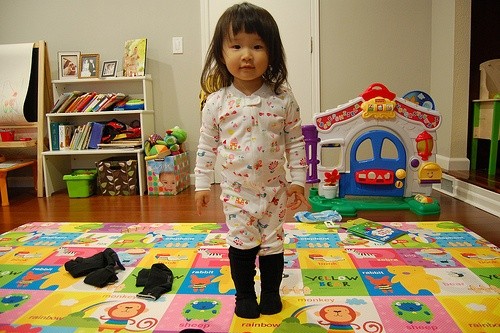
[126,47,140,76]
[158,170,175,194]
[192,1,312,320]
[63,62,76,75]
[82,58,95,76]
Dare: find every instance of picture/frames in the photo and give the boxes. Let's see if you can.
[78,53,100,78]
[57,50,82,80]
[101,60,118,77]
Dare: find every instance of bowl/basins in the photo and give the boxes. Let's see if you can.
[0,131,15,142]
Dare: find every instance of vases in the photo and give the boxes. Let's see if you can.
[322,184,339,199]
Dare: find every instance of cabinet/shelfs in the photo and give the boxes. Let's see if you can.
[41,73,154,197]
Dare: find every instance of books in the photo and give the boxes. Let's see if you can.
[347,222,409,245]
[50,88,144,114]
[339,217,371,230]
[50,121,142,152]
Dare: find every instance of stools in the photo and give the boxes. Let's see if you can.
[0,158,37,207]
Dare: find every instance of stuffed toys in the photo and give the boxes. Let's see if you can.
[144,125,187,160]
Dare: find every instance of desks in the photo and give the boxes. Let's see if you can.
[0,137,36,148]
[470,97,500,177]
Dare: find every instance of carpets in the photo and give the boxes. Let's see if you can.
[0,220,500,333]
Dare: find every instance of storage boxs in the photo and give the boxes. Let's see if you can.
[0,130,16,142]
[62,168,97,199]
[146,151,191,197]
[94,154,138,197]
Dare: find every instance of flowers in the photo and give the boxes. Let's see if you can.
[324,169,340,186]
